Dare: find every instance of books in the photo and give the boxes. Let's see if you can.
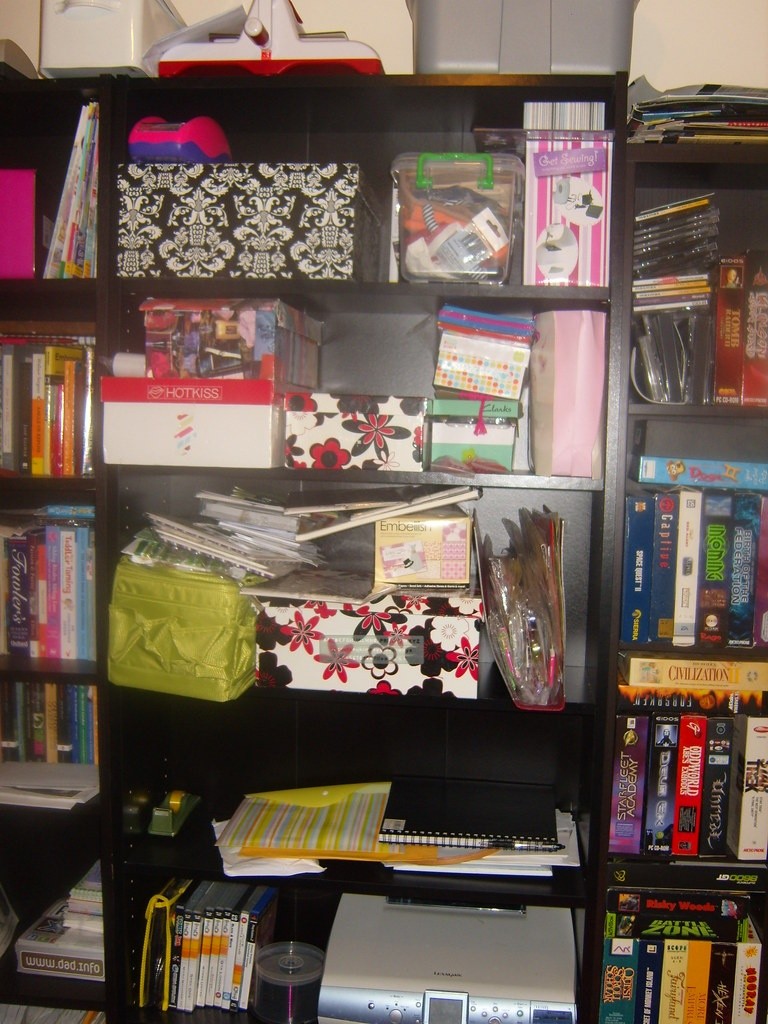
[0,505,96,661]
[0,678,97,765]
[120,489,322,585]
[619,487,768,692]
[167,880,277,1014]
[378,771,565,852]
[42,101,99,278]
[0,335,94,479]
[609,708,768,861]
[64,858,104,935]
[599,916,763,1024]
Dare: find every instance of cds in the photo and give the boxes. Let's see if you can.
[259,953,320,982]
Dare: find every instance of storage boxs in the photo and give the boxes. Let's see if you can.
[38,0,190,81]
[406,1,639,76]
[16,895,105,982]
[0,167,64,280]
[97,129,612,706]
[597,450,768,1024]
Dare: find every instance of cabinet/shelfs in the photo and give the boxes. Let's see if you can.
[95,68,619,1024]
[0,77,104,1024]
[615,140,767,589]
